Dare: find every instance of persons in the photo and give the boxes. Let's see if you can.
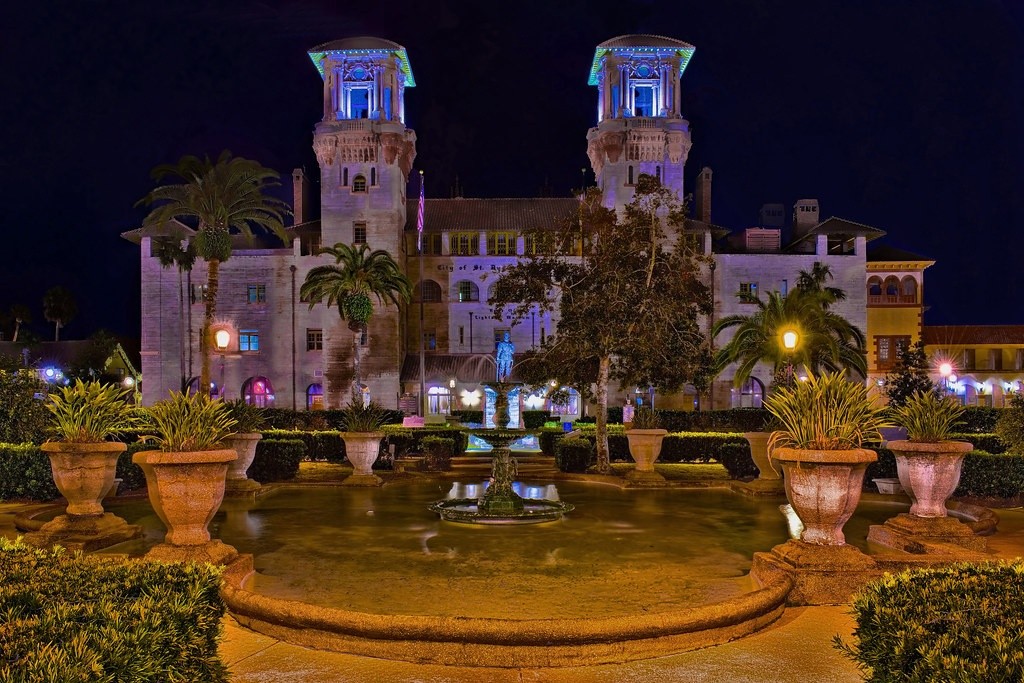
[496,331,515,382]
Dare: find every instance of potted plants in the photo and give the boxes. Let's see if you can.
[211,398,270,481]
[883,386,975,520]
[555,437,591,473]
[131,386,239,545]
[40,377,143,516]
[745,416,794,481]
[627,407,667,471]
[758,363,898,546]
[338,403,393,476]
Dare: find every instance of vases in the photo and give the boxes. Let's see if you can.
[873,479,902,495]
[106,479,123,497]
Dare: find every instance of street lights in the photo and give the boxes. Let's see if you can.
[780,328,800,393]
[123,377,134,405]
[212,327,231,403]
[44,367,56,426]
[938,363,953,397]
